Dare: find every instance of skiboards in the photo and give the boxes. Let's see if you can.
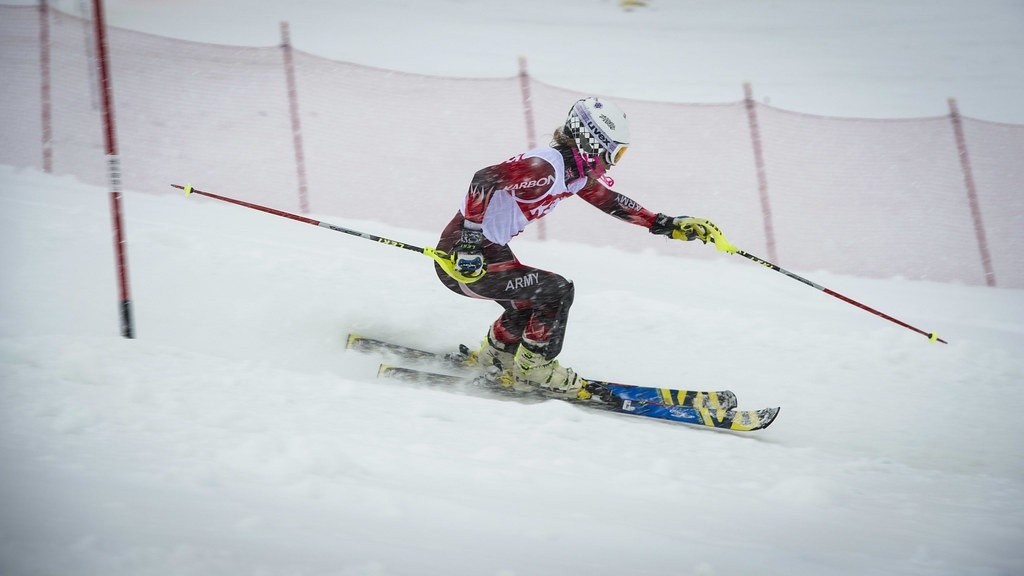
[347,332,780,431]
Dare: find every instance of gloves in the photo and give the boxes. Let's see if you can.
[649,213,711,242]
[453,228,483,277]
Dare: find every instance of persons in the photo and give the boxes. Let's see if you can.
[434,96,736,401]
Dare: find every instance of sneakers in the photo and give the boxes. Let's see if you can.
[477,322,583,399]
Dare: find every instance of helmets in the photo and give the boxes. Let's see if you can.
[565,96,631,163]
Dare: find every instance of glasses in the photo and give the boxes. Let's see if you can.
[611,143,629,166]
[605,150,613,165]
